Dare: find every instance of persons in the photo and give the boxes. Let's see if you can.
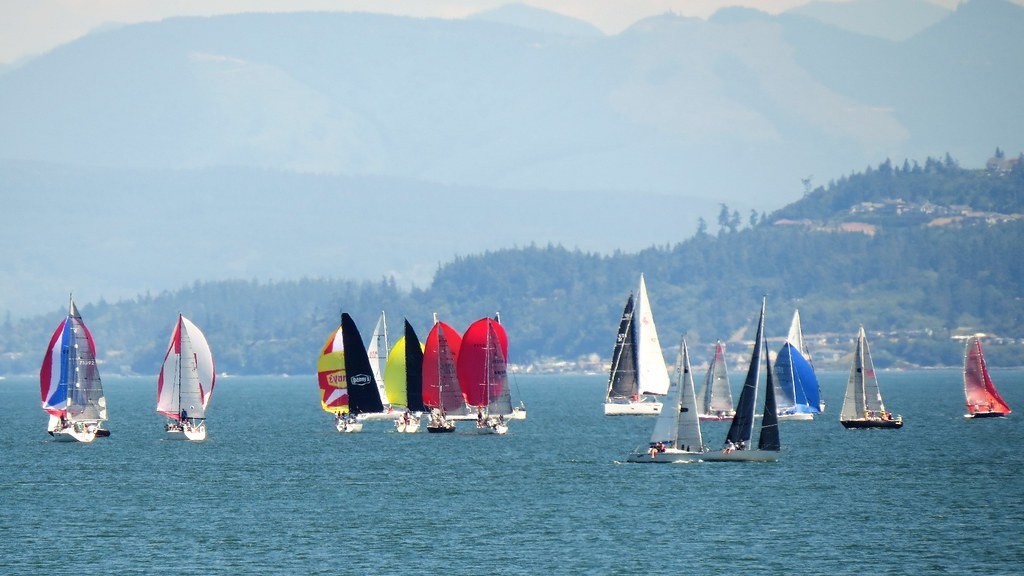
[975,399,994,412]
[865,410,903,425]
[334,411,358,424]
[477,406,505,432]
[59,413,94,434]
[629,393,638,403]
[164,409,192,432]
[648,410,747,459]
[387,404,455,429]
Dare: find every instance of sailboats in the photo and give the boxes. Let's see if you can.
[701,293,783,462]
[754,341,821,422]
[316,306,528,434]
[838,322,904,428]
[782,308,825,413]
[963,334,1013,420]
[696,339,737,420]
[38,292,109,444]
[602,272,670,416]
[628,335,708,464]
[157,312,217,444]
